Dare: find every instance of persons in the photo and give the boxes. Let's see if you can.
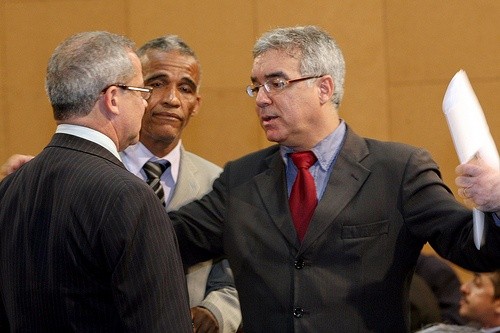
[0,30,194,333]
[411,251,500,333]
[115,33,242,333]
[0,25,500,333]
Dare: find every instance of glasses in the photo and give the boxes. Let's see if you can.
[246,74,324,98]
[101,83,154,99]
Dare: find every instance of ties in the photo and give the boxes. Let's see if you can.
[142,162,171,208]
[287,151,319,245]
